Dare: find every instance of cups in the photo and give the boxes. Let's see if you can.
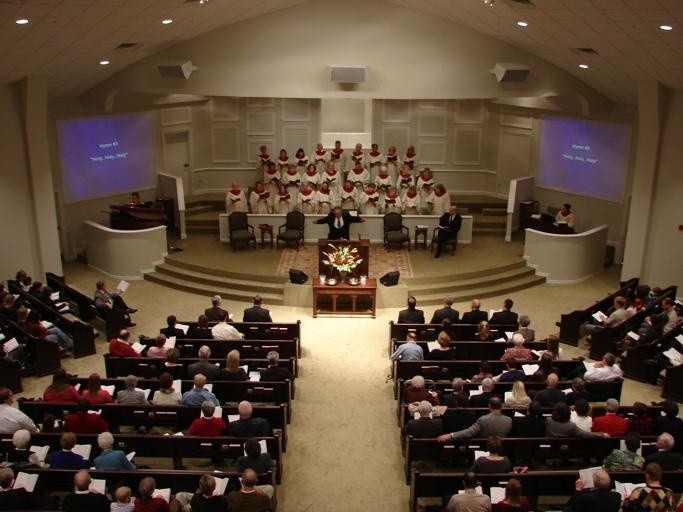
[319,275,326,286]
[360,275,366,286]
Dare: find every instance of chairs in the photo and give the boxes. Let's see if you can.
[381,211,411,254]
[428,226,458,256]
[273,210,306,252]
[227,210,257,253]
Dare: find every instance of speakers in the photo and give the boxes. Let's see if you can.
[494,62,532,83]
[156,61,197,81]
[329,64,368,84]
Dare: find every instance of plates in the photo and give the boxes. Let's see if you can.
[325,278,339,286]
[346,278,361,286]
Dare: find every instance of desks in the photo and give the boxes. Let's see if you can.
[258,224,274,249]
[414,226,428,250]
[311,271,379,321]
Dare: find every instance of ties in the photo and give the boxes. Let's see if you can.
[337,218,341,227]
[449,215,453,224]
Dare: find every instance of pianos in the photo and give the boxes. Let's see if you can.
[100,204,170,231]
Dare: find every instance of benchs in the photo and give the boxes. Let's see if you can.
[383,275,683,512]
[0,265,304,512]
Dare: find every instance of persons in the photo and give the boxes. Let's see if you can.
[312,206,366,241]
[434,205,462,258]
[225,141,450,214]
[129,191,145,204]
[390,286,683,511]
[1,270,293,512]
[555,204,575,229]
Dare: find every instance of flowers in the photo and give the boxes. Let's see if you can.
[320,239,364,278]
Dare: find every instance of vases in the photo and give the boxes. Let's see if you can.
[338,270,347,284]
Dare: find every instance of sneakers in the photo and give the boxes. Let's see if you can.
[125,307,138,327]
[433,253,441,258]
